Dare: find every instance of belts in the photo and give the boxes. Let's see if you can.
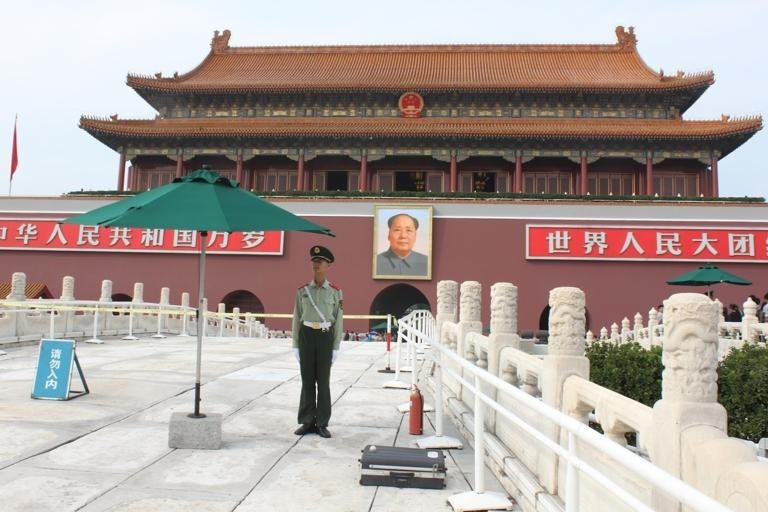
[304,321,332,330]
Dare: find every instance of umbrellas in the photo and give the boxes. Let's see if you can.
[370,321,397,330]
[667,261,752,298]
[55,165,336,415]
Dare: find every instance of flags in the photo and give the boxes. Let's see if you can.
[11,127,18,179]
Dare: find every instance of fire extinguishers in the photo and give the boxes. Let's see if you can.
[410,379,424,435]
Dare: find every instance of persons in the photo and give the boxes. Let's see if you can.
[705,291,768,342]
[291,244,343,437]
[657,304,664,323]
[377,214,428,276]
[267,328,287,339]
[344,330,397,343]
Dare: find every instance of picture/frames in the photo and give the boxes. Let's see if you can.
[372,205,433,282]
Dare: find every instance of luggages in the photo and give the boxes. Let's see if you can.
[357,445,447,489]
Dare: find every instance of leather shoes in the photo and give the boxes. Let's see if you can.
[317,427,331,438]
[294,422,315,435]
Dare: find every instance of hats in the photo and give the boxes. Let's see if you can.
[310,245,334,264]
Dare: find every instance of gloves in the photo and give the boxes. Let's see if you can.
[331,350,338,364]
[294,348,300,362]
